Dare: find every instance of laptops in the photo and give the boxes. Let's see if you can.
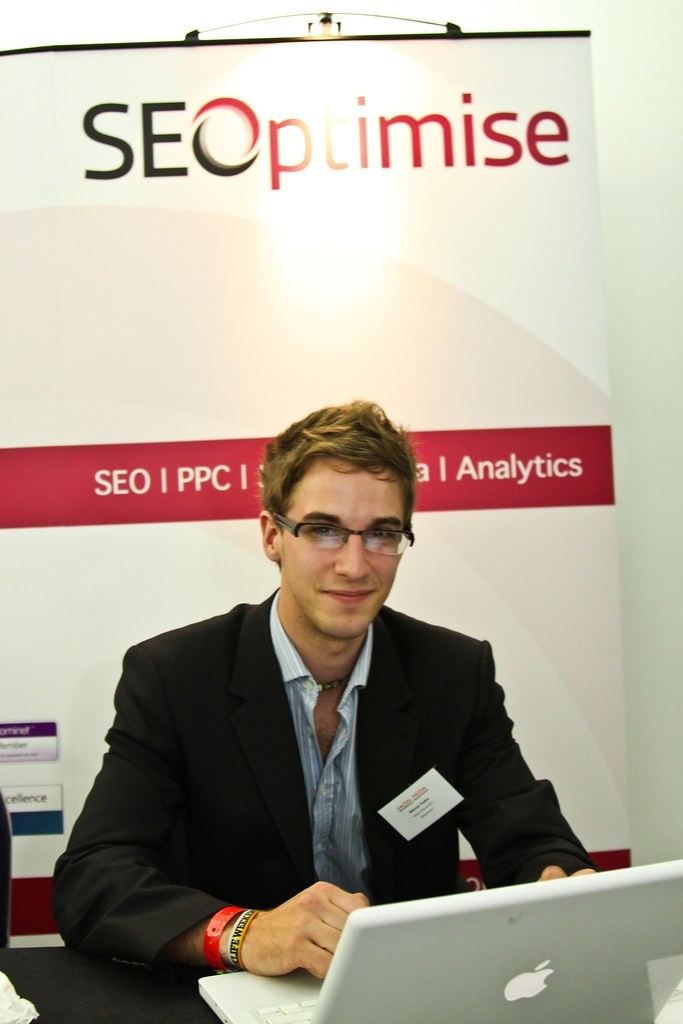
[196,859,683,1024]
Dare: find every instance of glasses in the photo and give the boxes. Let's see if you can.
[273,511,415,557]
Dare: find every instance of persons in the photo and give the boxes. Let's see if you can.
[49,401,601,989]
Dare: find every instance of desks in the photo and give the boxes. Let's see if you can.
[0,947,222,1024]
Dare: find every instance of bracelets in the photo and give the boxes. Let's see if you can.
[228,909,261,973]
[203,907,248,974]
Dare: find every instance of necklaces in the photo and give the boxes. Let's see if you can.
[318,677,346,691]
